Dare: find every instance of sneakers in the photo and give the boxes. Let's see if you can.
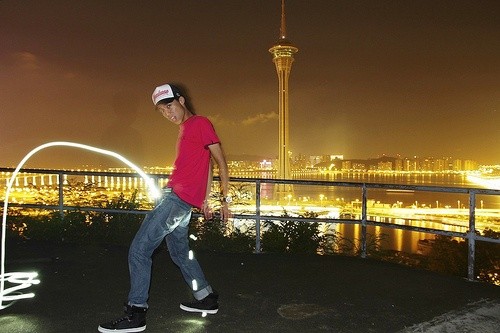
[180,293,218,313]
[98,303,147,332]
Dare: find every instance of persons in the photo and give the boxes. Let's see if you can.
[98,83,232,332]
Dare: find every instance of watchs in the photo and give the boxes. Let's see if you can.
[220,196,231,204]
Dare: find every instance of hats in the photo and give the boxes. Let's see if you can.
[152,83,183,110]
